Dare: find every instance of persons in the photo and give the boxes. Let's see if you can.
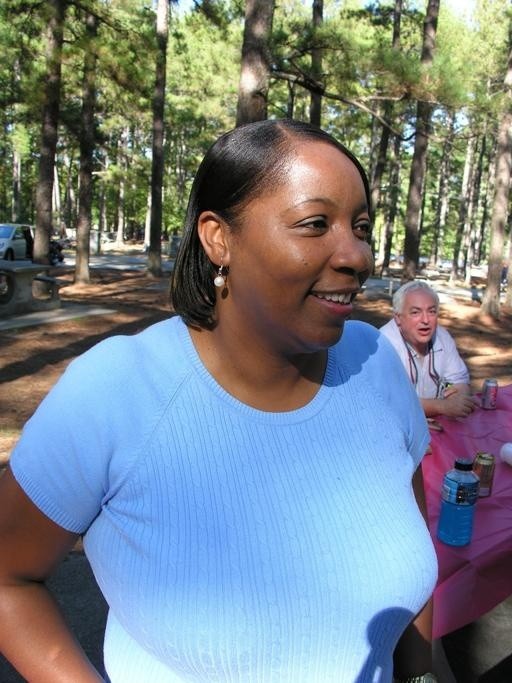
[378,280,480,418]
[0,119,483,683]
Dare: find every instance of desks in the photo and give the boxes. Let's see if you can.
[0,260,51,310]
[412,385,511,643]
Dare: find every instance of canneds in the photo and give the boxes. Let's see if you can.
[473,453,495,498]
[482,380,497,409]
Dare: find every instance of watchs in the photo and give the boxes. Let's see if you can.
[405,673,438,683]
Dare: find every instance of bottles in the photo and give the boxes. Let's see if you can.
[438,457,481,547]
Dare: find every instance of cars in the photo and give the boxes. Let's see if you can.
[0,222,34,259]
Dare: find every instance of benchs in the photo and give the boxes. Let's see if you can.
[32,275,70,310]
